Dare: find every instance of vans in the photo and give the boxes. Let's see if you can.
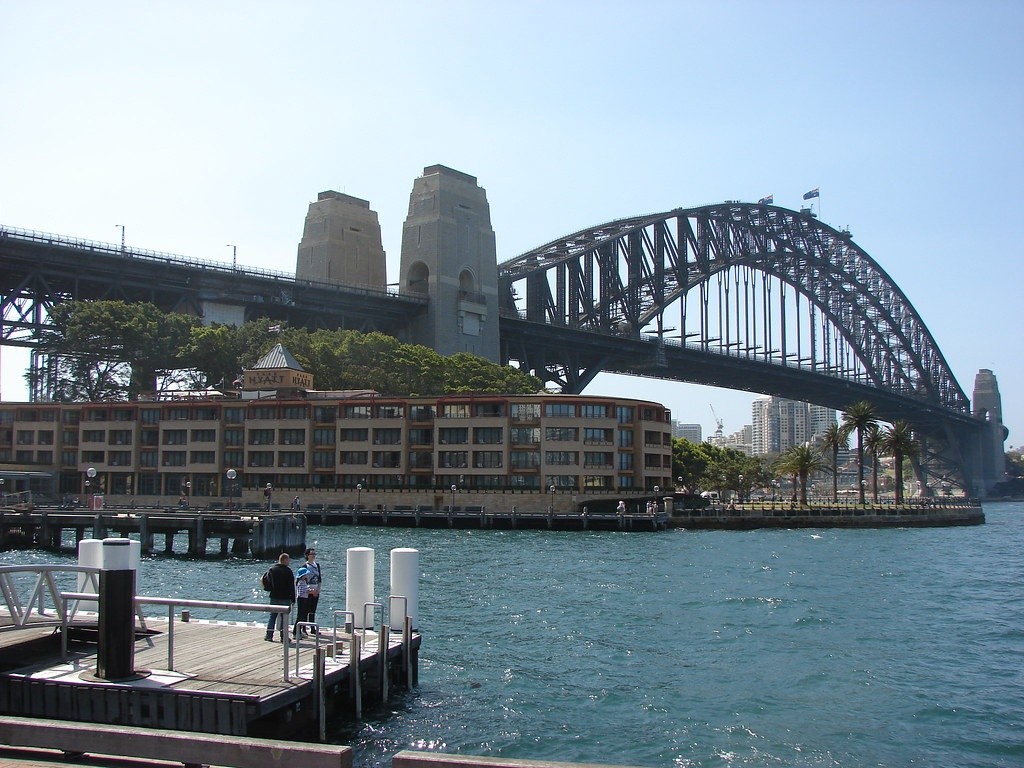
[700,491,720,504]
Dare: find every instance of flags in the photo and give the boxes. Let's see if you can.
[762,195,772,204]
[804,189,819,199]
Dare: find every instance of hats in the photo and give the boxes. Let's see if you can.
[296,568,310,578]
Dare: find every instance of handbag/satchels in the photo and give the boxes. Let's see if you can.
[260,567,274,591]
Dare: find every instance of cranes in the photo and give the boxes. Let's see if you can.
[709,403,724,430]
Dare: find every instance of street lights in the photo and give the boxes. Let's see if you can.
[771,479,777,510]
[926,482,933,507]
[227,468,236,515]
[861,479,869,509]
[87,467,97,511]
[550,485,555,508]
[654,486,660,506]
[738,475,744,516]
[266,483,272,515]
[915,480,921,506]
[356,483,362,505]
[84,480,90,508]
[186,481,192,510]
[451,484,457,512]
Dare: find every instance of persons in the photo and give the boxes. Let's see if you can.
[264,554,295,644]
[261,483,272,512]
[294,548,322,639]
[292,496,300,510]
[646,501,658,514]
[616,501,626,515]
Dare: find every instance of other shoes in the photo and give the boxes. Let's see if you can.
[292,634,302,640]
[311,630,321,635]
[265,638,275,642]
[281,637,291,642]
[301,632,308,638]
[302,630,308,635]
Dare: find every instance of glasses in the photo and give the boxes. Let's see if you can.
[308,553,316,556]
